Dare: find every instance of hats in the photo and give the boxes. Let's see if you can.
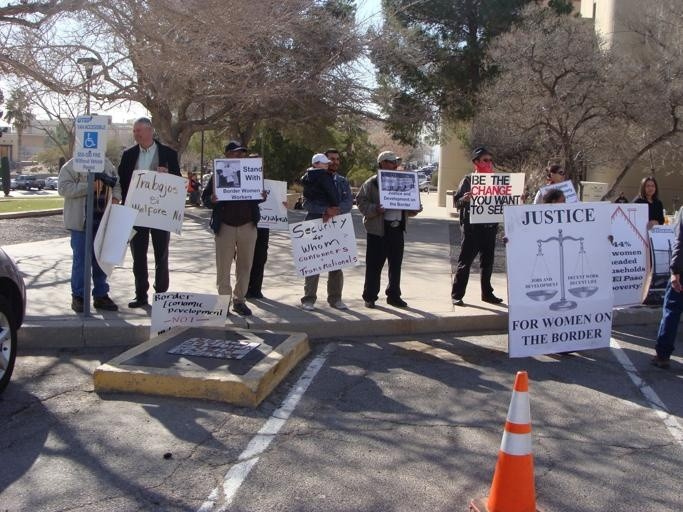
[472,147,494,160]
[226,142,248,153]
[311,153,333,165]
[378,150,402,165]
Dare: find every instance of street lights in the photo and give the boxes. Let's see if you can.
[74,54,102,116]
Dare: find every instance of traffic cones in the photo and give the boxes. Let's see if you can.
[467,367,544,512]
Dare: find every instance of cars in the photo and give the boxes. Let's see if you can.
[410,160,438,191]
[0,246,30,402]
[0,172,58,192]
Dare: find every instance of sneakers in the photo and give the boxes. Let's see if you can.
[71,294,84,313]
[94,296,117,311]
[302,302,315,311]
[648,355,670,367]
[335,303,347,308]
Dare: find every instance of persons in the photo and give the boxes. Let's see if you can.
[295,147,354,312]
[294,198,302,209]
[613,191,628,203]
[200,142,266,316]
[116,117,182,308]
[532,164,566,205]
[649,204,683,368]
[354,151,423,309]
[186,173,202,205]
[629,175,664,226]
[56,155,119,313]
[500,188,566,249]
[243,199,291,298]
[298,153,340,207]
[449,146,530,306]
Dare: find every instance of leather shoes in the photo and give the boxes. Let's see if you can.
[452,298,463,305]
[387,298,407,307]
[127,296,148,308]
[483,295,503,304]
[233,304,252,315]
[246,291,263,298]
[364,300,374,308]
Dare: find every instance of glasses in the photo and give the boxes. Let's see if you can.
[557,170,563,174]
[389,160,400,164]
[481,158,493,162]
